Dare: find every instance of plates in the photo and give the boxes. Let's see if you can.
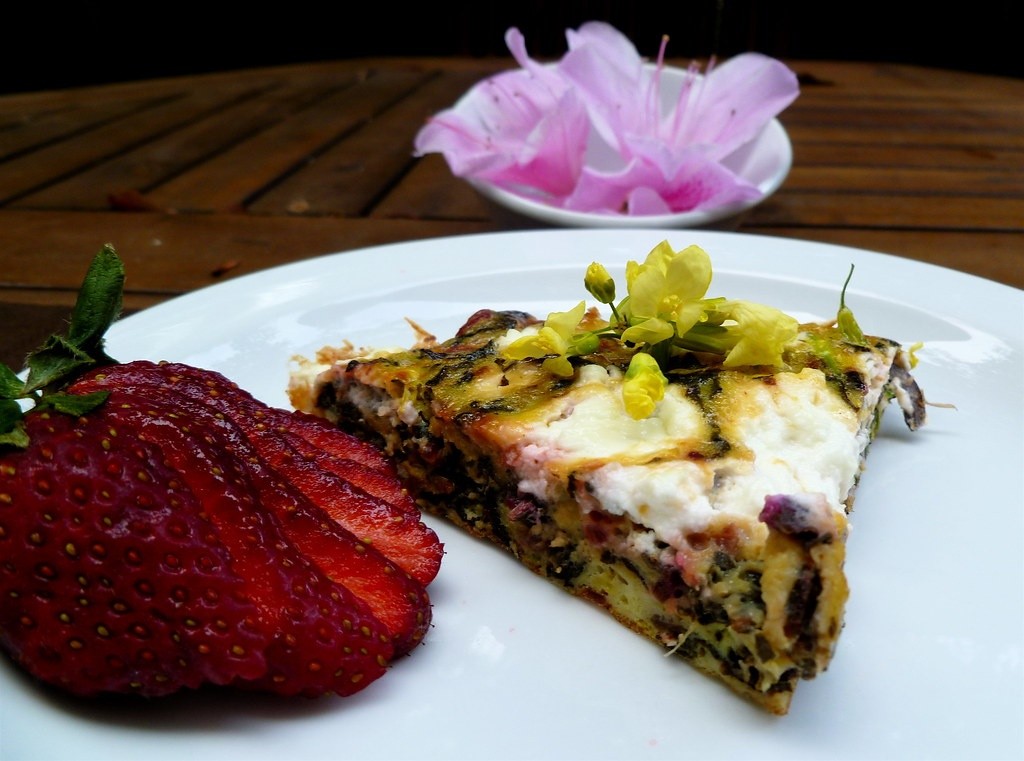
[0,230,1024,761]
[450,62,794,230]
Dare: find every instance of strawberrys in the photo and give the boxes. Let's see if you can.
[0,246,442,713]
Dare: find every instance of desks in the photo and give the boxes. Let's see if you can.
[0,62,1024,761]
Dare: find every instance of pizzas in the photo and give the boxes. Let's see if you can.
[309,245,936,713]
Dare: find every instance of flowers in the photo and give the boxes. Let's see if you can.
[413,22,803,214]
